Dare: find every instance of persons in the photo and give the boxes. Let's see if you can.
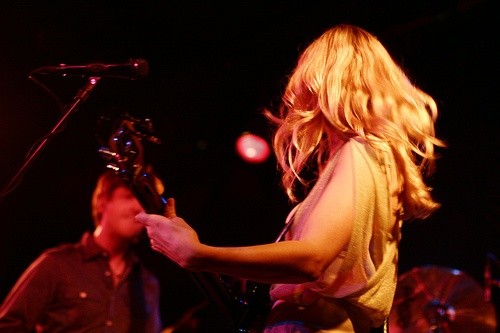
[0,168,165,333]
[133,24,445,333]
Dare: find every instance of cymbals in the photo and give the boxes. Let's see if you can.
[387,265,496,332]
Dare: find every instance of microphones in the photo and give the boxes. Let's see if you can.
[32,58,149,85]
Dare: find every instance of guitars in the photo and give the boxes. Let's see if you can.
[97,111,270,332]
